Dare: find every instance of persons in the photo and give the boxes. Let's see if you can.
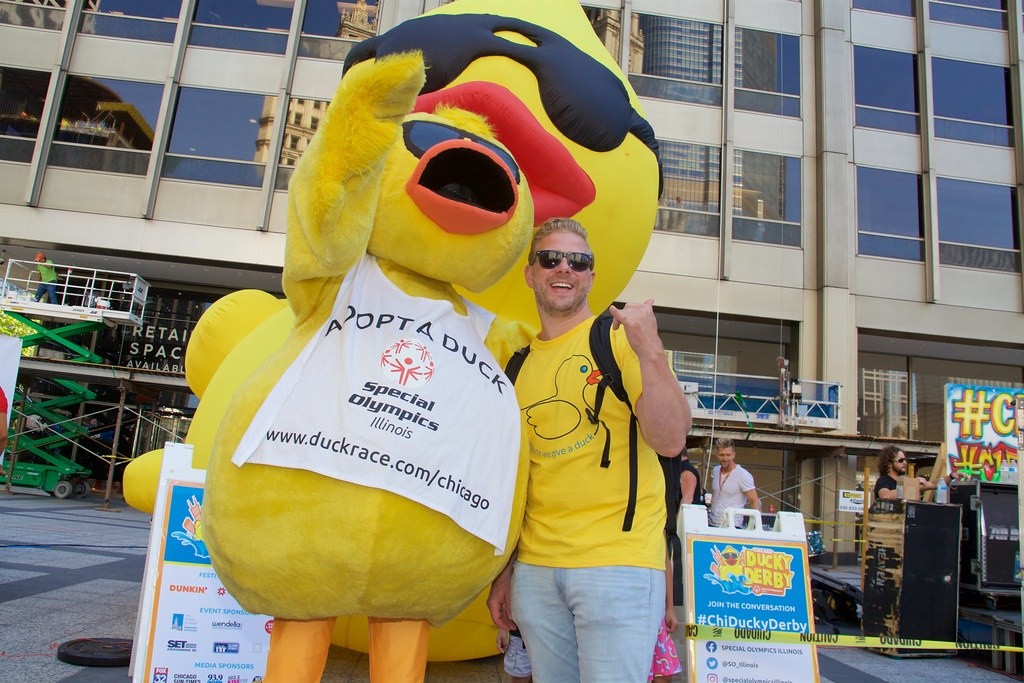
[0,388,9,484]
[676,439,703,519]
[874,445,969,501]
[499,215,699,683]
[645,529,682,683]
[708,437,761,530]
[496,608,533,681]
[31,253,60,305]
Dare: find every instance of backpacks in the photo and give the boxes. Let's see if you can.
[504,307,683,537]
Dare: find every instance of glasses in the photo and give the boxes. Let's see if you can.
[531,250,592,272]
[888,458,907,462]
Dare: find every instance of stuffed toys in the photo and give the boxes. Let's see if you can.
[199,50,536,683]
